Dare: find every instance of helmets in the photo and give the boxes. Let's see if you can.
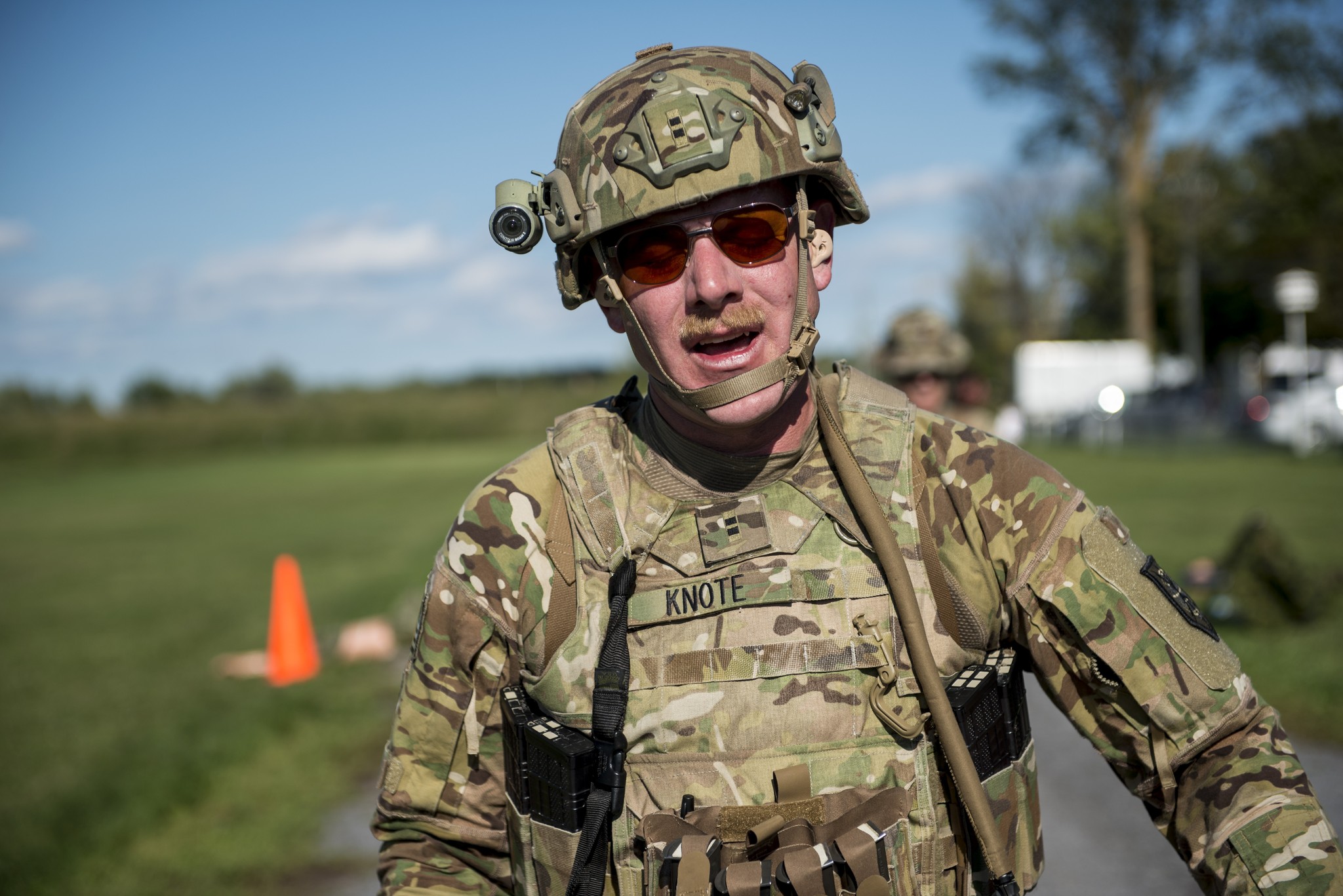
[543,45,870,312]
[872,311,972,382]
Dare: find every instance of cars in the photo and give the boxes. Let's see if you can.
[1246,369,1337,455]
[1061,382,1256,449]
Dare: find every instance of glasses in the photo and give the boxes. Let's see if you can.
[603,200,798,288]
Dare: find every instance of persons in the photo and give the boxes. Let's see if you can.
[875,306,977,418]
[365,36,1342,896]
